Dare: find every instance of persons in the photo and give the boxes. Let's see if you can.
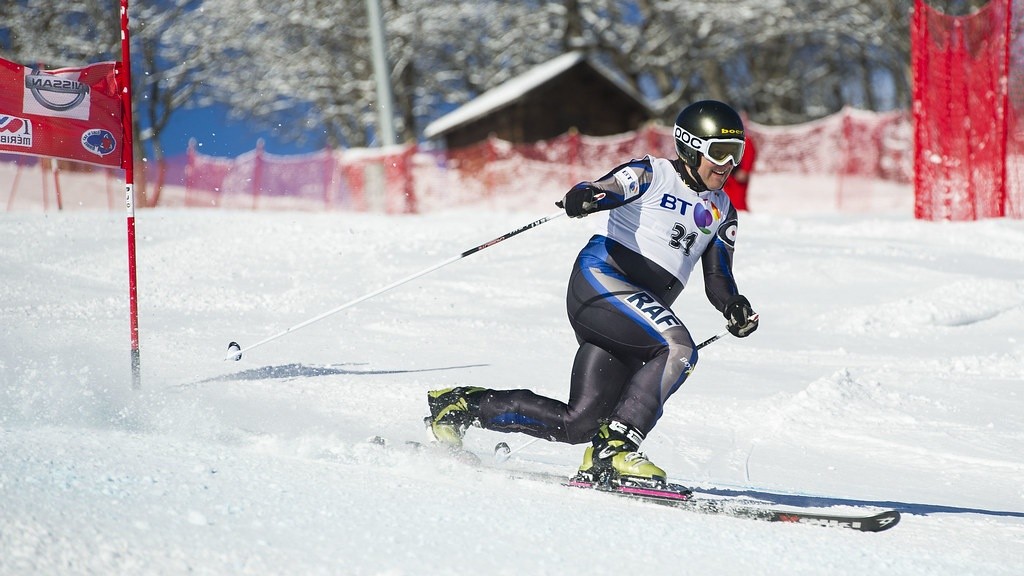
[723,109,757,212]
[426,100,757,490]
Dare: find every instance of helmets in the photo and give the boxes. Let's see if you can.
[675,100,745,168]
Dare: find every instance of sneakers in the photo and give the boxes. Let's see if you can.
[579,421,666,486]
[427,385,487,448]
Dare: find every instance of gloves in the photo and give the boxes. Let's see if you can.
[562,187,598,219]
[724,304,759,337]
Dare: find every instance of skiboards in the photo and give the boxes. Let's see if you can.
[365,434,902,534]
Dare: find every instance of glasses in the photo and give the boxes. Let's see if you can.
[704,139,746,167]
